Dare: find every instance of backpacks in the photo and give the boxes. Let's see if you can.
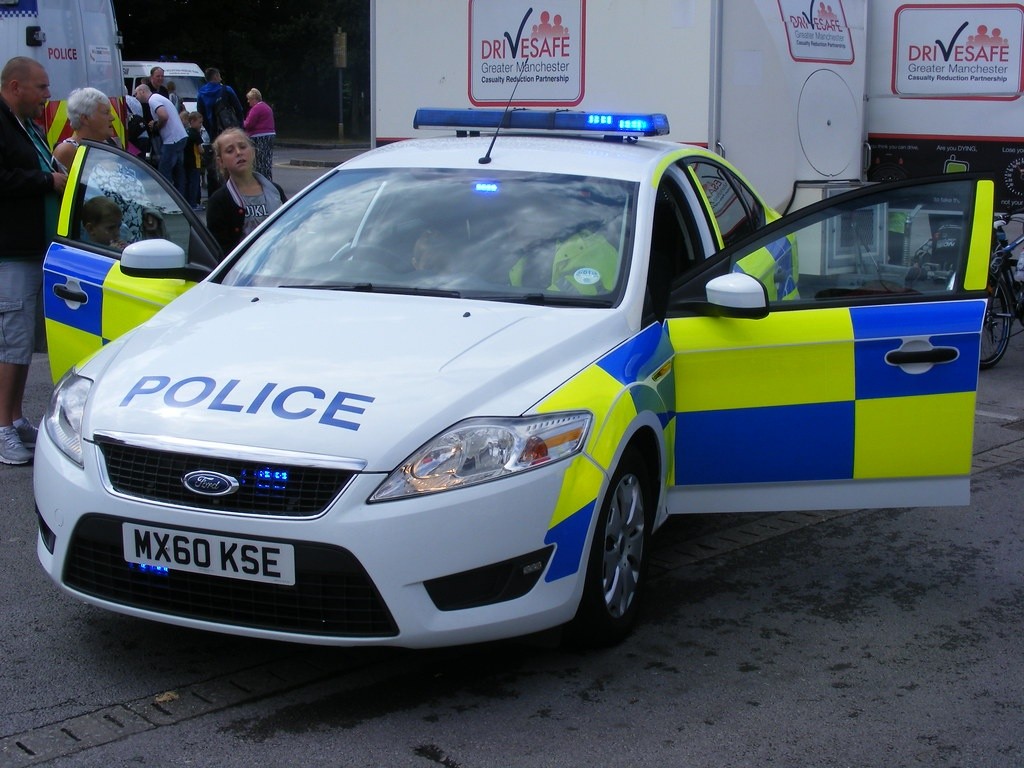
[210,86,242,135]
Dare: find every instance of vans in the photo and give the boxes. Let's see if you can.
[121,57,211,143]
[0,0,129,152]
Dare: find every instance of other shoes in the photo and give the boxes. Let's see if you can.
[193,204,206,212]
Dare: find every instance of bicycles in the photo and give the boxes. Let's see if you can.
[933,206,1024,370]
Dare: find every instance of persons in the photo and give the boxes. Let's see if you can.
[119,65,217,210]
[204,127,287,255]
[412,228,456,272]
[53,88,168,245]
[243,88,277,180]
[0,55,78,464]
[506,208,619,296]
[195,68,245,133]
[83,196,128,250]
[887,212,911,266]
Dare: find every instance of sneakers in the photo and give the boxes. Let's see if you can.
[13,415,39,448]
[0,426,34,465]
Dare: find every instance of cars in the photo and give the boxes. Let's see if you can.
[31,110,998,647]
